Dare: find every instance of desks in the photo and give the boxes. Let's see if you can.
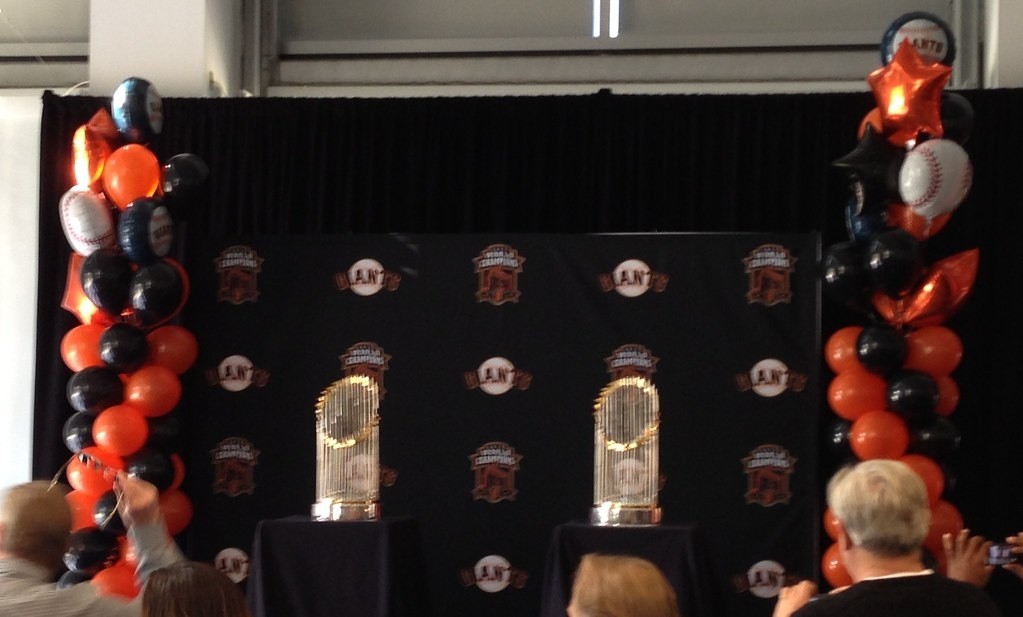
[249,511,425,617]
[542,520,715,617]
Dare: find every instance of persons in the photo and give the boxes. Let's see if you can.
[142,561,252,617]
[0,470,187,617]
[772,458,1023,617]
[566,554,678,617]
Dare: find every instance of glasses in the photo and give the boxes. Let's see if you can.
[46,452,131,529]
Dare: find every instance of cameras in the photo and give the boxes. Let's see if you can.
[985,542,1020,567]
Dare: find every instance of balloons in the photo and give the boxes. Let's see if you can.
[818,43,988,584]
[58,77,206,599]
